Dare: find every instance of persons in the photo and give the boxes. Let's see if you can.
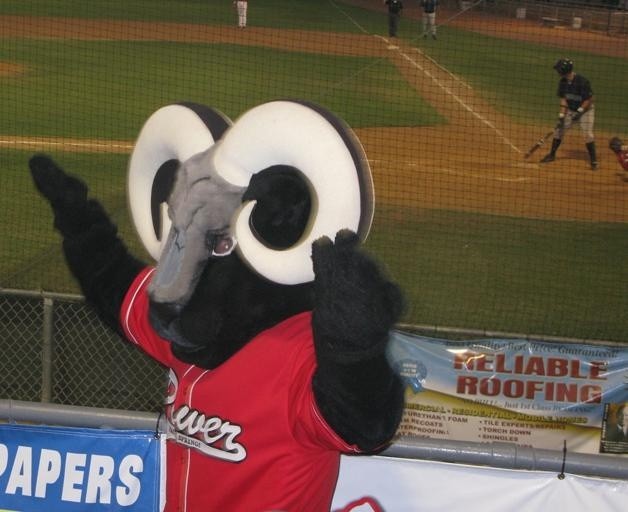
[538,57,602,170]
[420,0,440,40]
[608,404,628,442]
[235,1,248,28]
[384,0,403,38]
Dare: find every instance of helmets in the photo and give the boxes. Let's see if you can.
[553,57,573,73]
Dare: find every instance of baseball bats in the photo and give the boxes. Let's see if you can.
[524,127,559,159]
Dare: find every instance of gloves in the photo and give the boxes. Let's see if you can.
[556,107,584,129]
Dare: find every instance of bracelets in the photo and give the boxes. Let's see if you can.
[577,106,584,114]
[559,113,566,119]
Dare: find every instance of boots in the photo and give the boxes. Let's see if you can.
[585,141,598,170]
[540,138,561,163]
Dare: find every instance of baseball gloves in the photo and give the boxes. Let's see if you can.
[609,137,624,154]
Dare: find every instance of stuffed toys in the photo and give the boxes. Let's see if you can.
[26,98,409,511]
